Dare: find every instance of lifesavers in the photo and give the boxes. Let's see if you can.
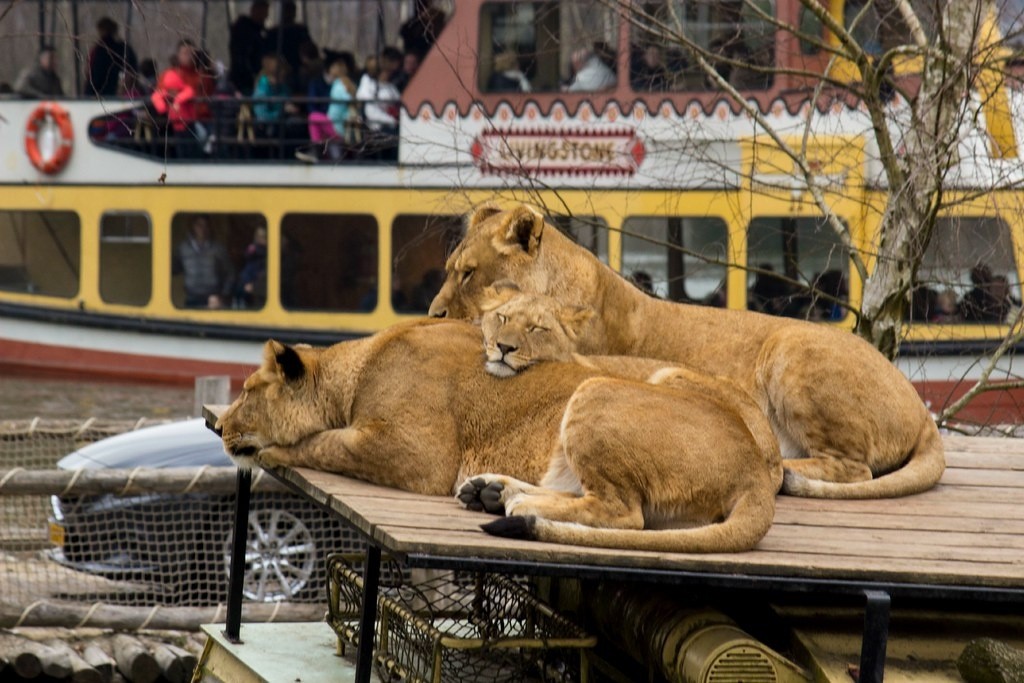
[22,100,76,177]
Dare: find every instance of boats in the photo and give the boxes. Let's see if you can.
[1,1,1023,445]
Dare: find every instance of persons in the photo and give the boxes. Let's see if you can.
[11,2,448,162]
[902,264,1018,326]
[629,262,823,322]
[485,27,776,94]
[177,213,269,312]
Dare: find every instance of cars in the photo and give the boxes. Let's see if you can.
[42,412,415,606]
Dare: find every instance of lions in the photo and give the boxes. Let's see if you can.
[214,203,947,555]
